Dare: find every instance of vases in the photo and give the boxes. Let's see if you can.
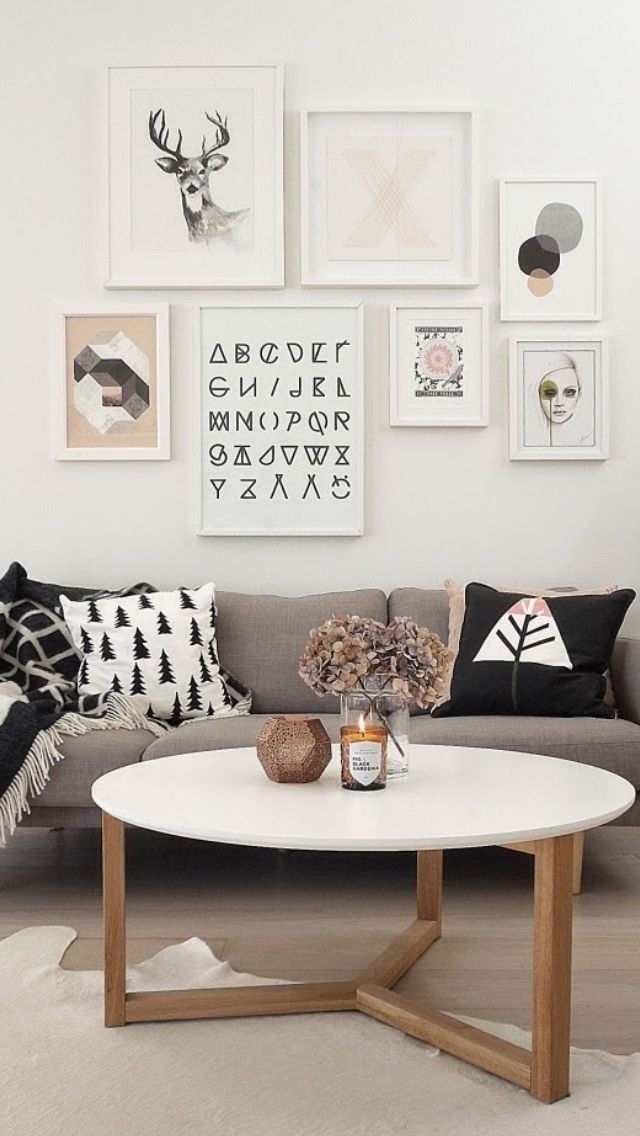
[342,662,410,776]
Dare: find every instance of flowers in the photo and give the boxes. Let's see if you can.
[296,604,453,718]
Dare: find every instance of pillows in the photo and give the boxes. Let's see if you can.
[441,581,622,717]
[59,582,233,723]
[467,585,625,721]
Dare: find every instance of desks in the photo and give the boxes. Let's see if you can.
[90,743,635,1105]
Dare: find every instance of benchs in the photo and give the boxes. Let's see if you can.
[0,590,640,849]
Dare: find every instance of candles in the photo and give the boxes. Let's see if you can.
[339,727,389,788]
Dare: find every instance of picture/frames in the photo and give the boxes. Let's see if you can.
[196,300,366,539]
[511,337,609,460]
[55,303,172,460]
[95,60,286,291]
[498,176,603,324]
[393,303,491,430]
[301,106,482,288]
